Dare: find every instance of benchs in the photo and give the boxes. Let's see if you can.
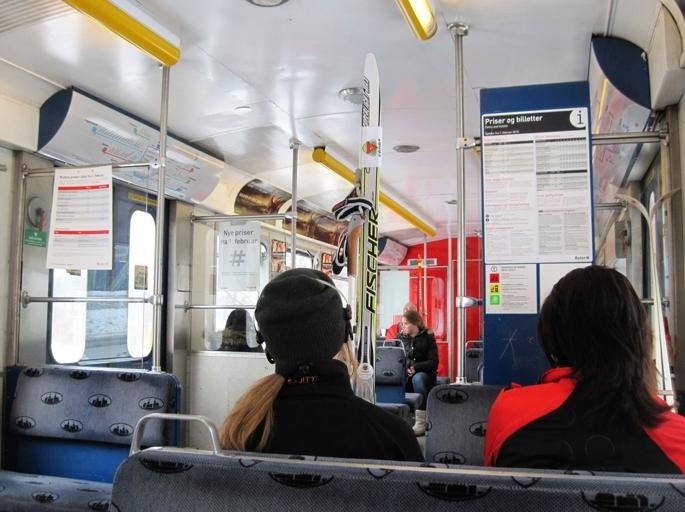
[109,412,677,510]
[424,385,510,464]
[2,362,182,512]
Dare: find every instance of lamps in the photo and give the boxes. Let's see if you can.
[62,0,181,70]
[395,0,439,42]
[312,143,439,240]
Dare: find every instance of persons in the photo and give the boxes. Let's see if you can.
[217,308,265,352]
[394,309,440,438]
[482,260,685,474]
[214,265,427,462]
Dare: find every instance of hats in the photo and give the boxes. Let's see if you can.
[254,268,347,365]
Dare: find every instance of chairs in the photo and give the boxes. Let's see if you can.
[465,338,483,382]
[372,337,423,429]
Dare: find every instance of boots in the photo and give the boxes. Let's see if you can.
[411,410,426,437]
